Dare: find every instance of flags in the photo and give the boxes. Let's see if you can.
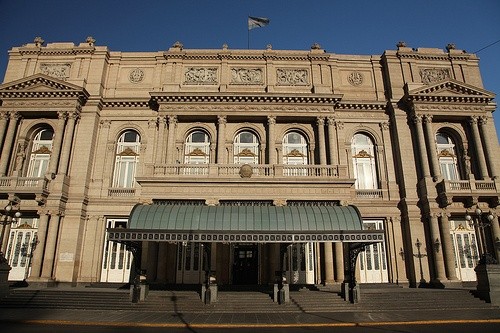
[248,15,271,31]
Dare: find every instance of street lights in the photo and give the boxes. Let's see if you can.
[465,203,494,266]
[0,201,21,270]
